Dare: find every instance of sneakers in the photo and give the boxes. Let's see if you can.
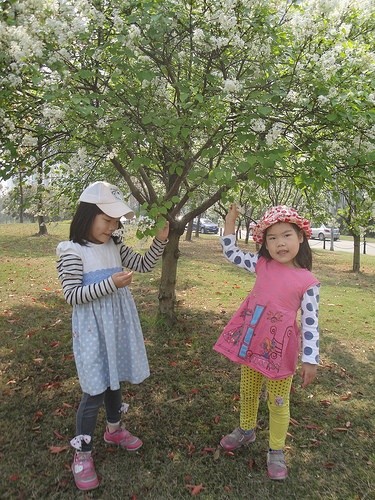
[71,453,99,490]
[104,426,143,450]
[220,428,256,450]
[267,451,288,479]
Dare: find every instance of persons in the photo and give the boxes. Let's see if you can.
[213,202,321,480]
[56,181,169,490]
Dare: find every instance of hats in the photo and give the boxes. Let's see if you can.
[253,205,312,245]
[79,181,134,219]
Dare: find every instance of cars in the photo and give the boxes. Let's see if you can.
[248,220,260,236]
[309,222,340,241]
[192,217,219,234]
